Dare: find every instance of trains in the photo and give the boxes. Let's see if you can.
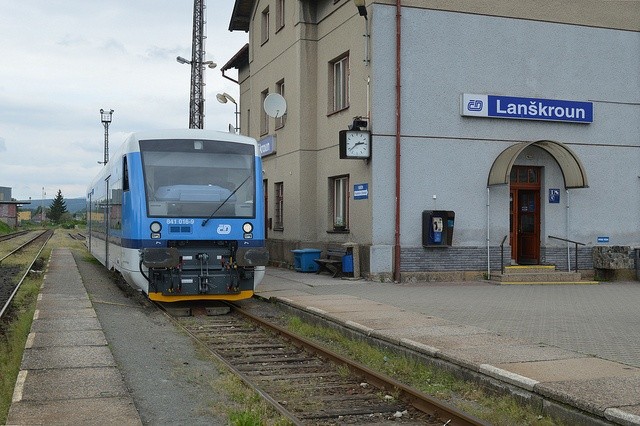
[85,128,269,303]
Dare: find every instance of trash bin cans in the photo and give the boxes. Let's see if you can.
[289,249,302,272]
[301,248,323,272]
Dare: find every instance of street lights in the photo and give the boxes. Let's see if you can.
[216,92,240,134]
[176,56,217,128]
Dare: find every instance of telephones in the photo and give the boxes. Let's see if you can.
[432,217,443,232]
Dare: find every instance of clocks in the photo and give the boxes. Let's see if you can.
[339,130,372,160]
